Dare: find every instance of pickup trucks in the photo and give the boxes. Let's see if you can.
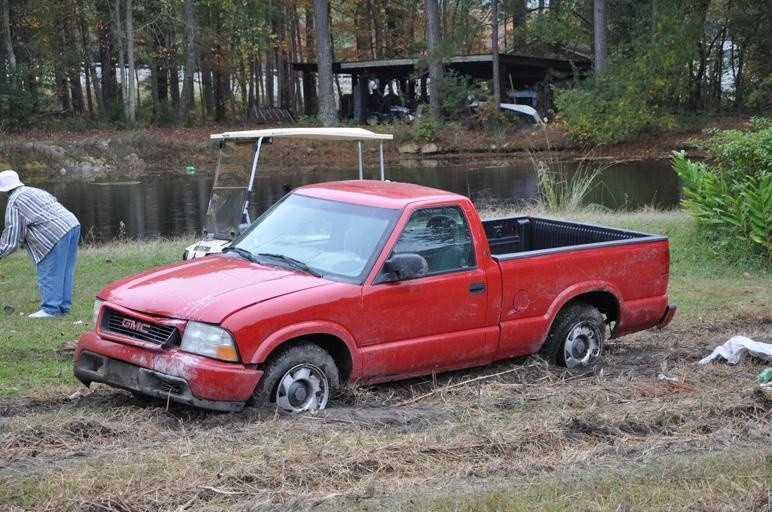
[72,175,679,427]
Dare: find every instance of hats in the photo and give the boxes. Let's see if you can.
[0,168,25,193]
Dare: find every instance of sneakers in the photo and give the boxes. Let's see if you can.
[28,309,57,319]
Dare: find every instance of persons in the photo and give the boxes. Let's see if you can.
[0,169,81,319]
